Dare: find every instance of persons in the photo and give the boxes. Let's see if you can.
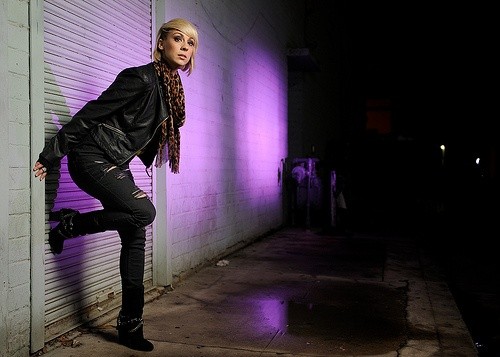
[33,17,199,352]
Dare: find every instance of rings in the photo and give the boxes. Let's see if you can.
[42,167,47,173]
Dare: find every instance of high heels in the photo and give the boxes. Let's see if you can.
[116,310,155,351]
[47,208,79,255]
